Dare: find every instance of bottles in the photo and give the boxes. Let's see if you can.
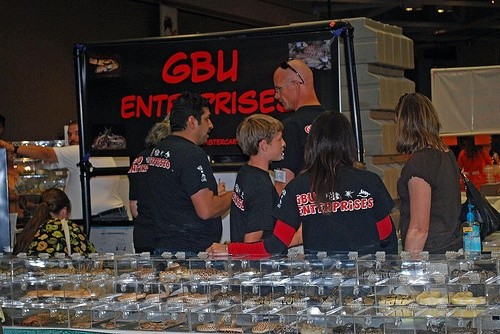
[462,204,482,262]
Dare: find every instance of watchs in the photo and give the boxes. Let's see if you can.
[13,143,20,153]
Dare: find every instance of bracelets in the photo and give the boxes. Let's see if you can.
[224,241,230,255]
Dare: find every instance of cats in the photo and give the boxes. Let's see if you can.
[92,127,126,151]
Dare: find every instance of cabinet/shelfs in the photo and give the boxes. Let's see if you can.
[12,141,65,229]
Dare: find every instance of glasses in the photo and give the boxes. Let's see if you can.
[273,60,305,84]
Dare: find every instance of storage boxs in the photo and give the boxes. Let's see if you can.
[0,251,500,334]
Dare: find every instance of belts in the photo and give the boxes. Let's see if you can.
[91,206,127,219]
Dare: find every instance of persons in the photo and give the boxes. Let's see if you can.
[144,94,234,256]
[273,59,329,196]
[205,110,398,258]
[394,93,480,253]
[0,120,84,233]
[451,133,500,192]
[11,188,100,257]
[229,114,287,243]
[128,112,174,255]
[0,137,28,219]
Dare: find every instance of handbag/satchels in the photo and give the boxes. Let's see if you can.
[458,167,500,242]
